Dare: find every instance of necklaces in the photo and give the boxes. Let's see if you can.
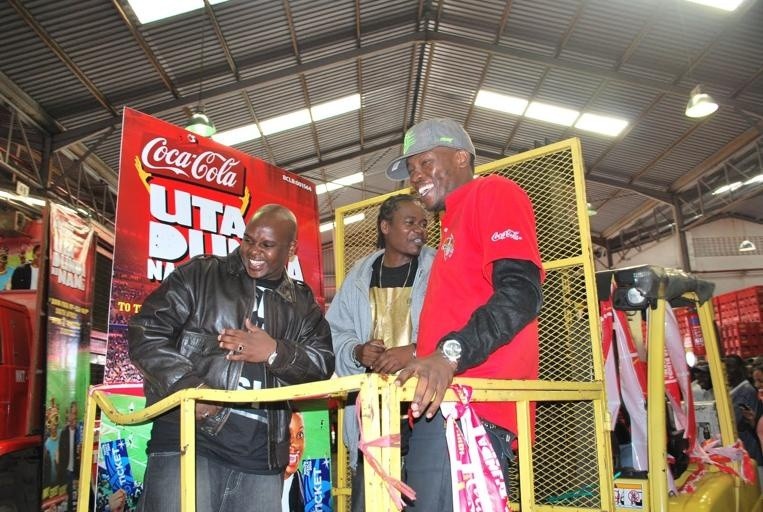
[379,255,413,313]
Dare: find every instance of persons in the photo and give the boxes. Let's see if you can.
[325,194,439,512]
[385,117,547,512]
[44,402,83,487]
[128,203,334,512]
[281,408,305,512]
[690,354,763,494]
[0,245,40,291]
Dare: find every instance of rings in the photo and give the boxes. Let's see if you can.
[238,345,243,353]
[202,411,209,418]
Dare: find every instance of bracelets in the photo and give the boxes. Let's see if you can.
[412,343,418,359]
[353,347,362,368]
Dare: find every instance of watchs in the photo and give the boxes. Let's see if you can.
[266,342,279,367]
[438,339,461,369]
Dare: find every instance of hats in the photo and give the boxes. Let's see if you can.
[385,118,475,181]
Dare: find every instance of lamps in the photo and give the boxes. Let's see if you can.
[675,2,718,118]
[587,201,596,217]
[182,8,215,138]
[737,240,756,253]
